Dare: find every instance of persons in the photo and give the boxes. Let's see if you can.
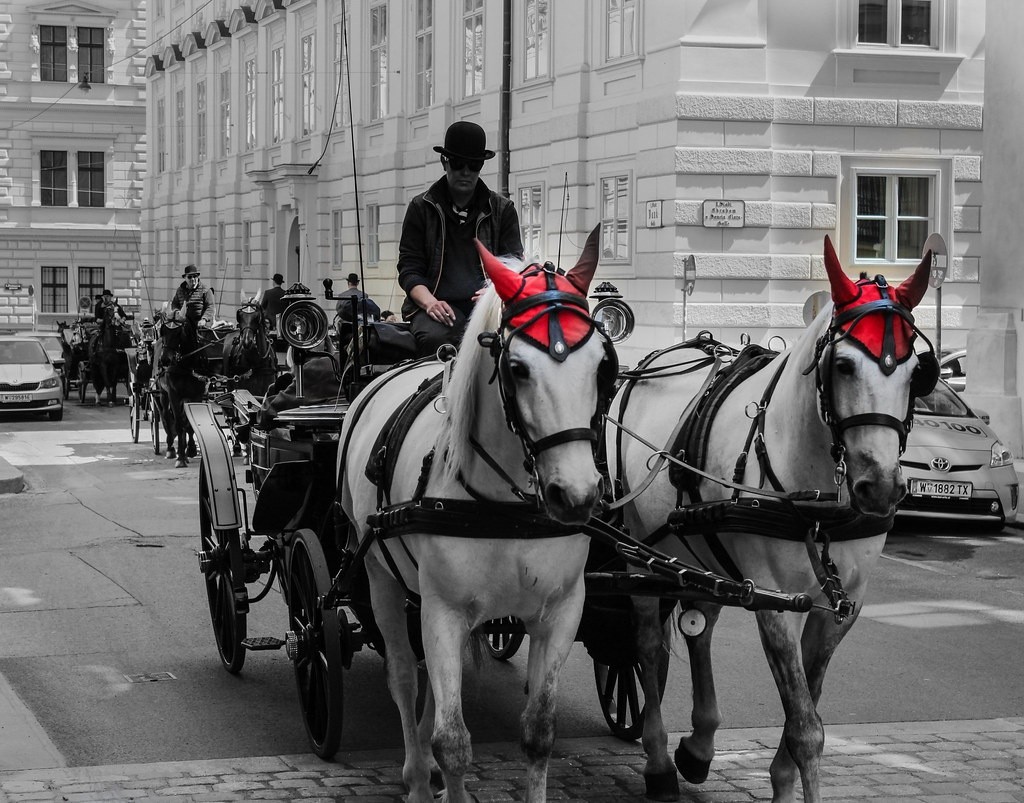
[397,121,524,360]
[171,265,215,328]
[95,290,127,331]
[336,274,368,318]
[261,274,290,330]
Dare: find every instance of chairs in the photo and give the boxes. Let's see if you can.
[367,320,419,377]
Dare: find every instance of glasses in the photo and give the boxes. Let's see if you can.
[185,275,198,279]
[441,153,485,172]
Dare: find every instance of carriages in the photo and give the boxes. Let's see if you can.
[61,296,140,407]
[183,220,942,803]
[125,287,279,468]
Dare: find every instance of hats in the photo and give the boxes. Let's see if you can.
[100,290,113,298]
[182,266,200,278]
[271,274,285,283]
[346,274,360,283]
[433,120,496,160]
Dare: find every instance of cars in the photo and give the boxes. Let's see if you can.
[14,332,67,397]
[0,336,67,421]
[890,376,1020,532]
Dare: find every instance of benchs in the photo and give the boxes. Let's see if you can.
[267,356,347,409]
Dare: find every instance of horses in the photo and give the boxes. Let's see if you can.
[332,220,936,803]
[55,288,279,469]
